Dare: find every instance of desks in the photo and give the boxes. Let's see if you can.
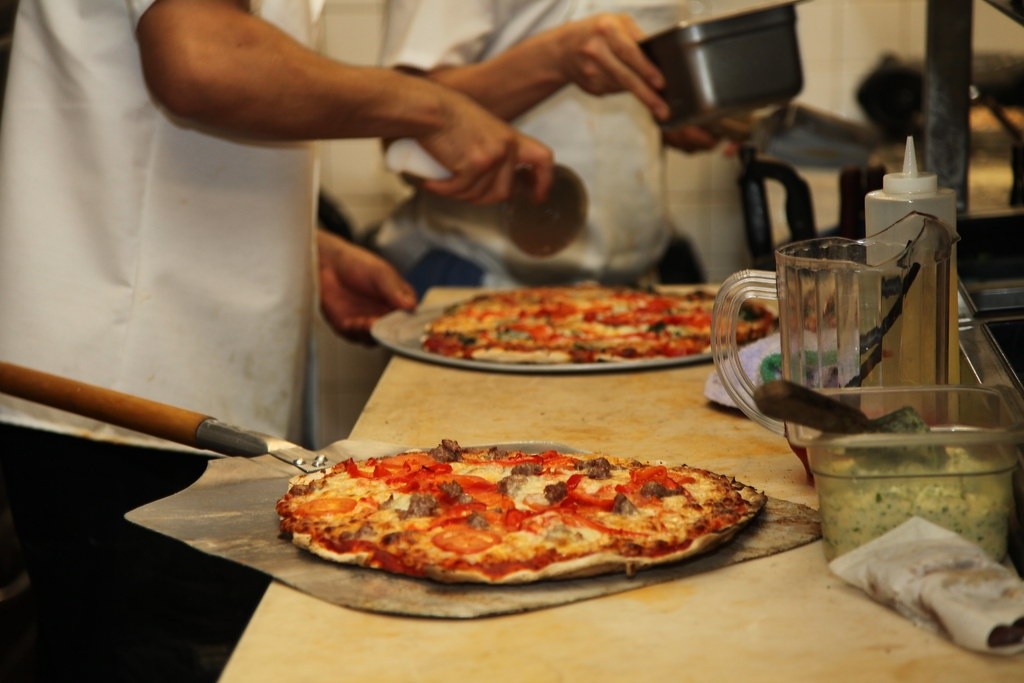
[217,282,1024,683]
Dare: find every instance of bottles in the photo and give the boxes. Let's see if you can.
[865,136,960,428]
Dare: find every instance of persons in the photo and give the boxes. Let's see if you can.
[0,0,553,683]
[378,0,799,306]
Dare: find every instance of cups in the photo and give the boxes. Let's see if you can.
[409,158,588,258]
[710,235,905,483]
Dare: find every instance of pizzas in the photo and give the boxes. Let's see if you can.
[421,284,772,364]
[275,439,768,584]
